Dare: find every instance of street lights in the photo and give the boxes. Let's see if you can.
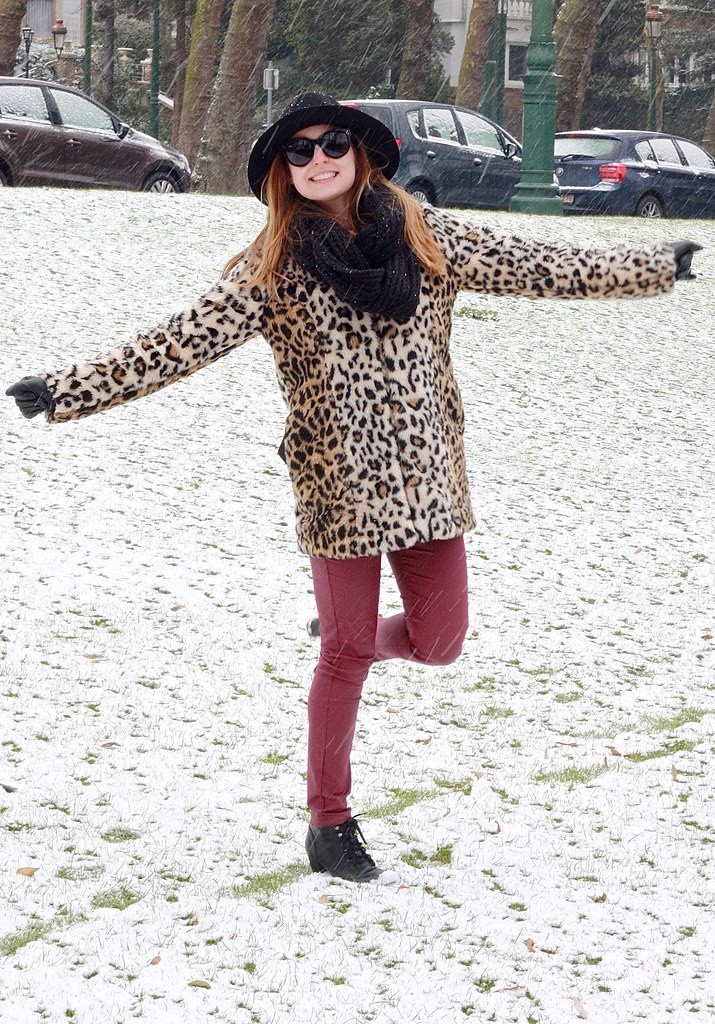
[22,23,35,78]
[643,4,666,131]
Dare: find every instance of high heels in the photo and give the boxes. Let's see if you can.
[305,812,384,882]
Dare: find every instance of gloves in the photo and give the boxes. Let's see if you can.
[670,241,703,281]
[4,376,51,419]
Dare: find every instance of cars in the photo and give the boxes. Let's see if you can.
[0,77,193,193]
[334,98,523,209]
[554,129,715,221]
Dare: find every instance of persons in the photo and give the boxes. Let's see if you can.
[5,92,704,884]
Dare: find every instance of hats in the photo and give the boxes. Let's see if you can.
[247,93,400,206]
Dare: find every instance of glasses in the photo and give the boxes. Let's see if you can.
[282,128,351,168]
